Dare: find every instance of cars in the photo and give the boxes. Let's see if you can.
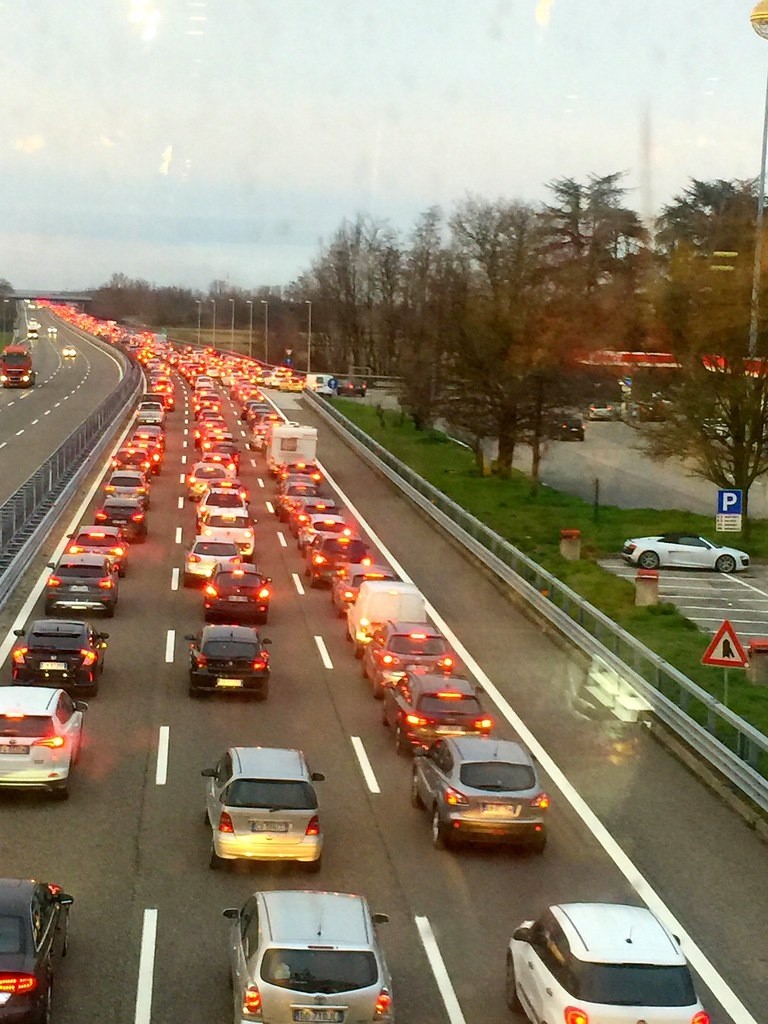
[0,685,89,800]
[24,300,495,761]
[410,734,551,854]
[221,889,397,1023]
[505,901,711,1024]
[700,418,729,440]
[0,876,75,1024]
[582,402,614,421]
[201,746,328,873]
[551,418,585,443]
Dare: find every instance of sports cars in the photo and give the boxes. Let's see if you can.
[620,530,751,574]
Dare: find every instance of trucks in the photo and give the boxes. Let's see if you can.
[0,345,37,389]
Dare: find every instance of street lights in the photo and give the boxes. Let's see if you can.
[210,300,216,348]
[229,299,235,352]
[246,301,253,358]
[304,300,312,372]
[195,301,202,345]
[261,300,268,366]
